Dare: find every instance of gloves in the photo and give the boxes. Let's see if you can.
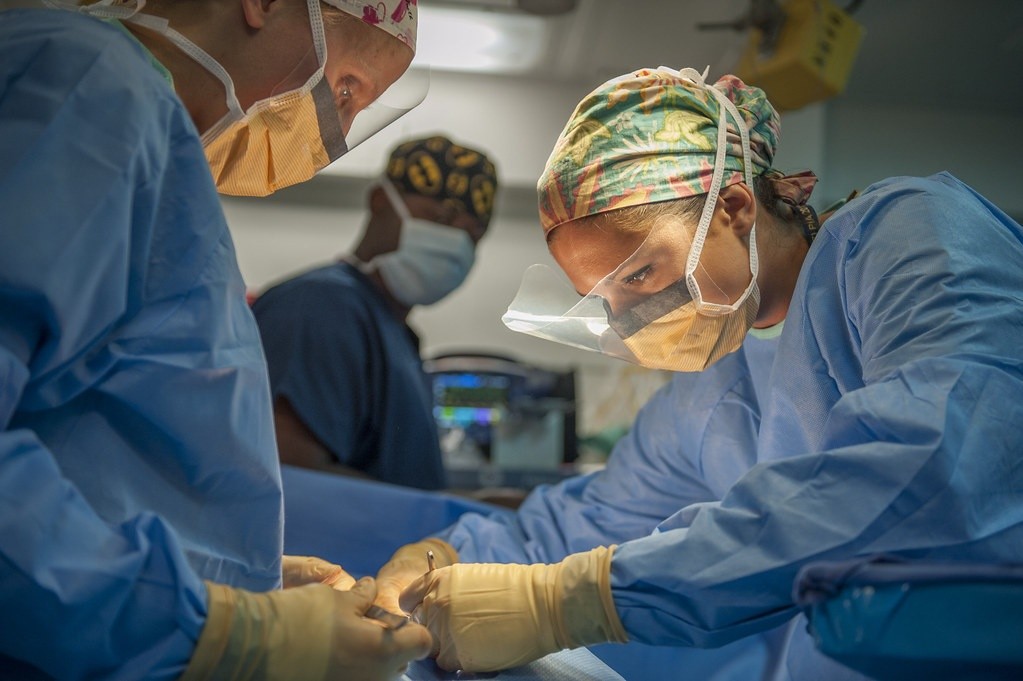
[372,538,457,616]
[399,545,628,672]
[187,581,433,681]
[282,554,356,591]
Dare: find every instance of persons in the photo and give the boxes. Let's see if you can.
[0,0,430,680]
[249,134,527,512]
[372,67,1023,681]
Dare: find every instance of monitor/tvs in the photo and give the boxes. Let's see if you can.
[428,371,511,451]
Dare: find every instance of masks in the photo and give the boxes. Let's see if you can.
[370,177,477,307]
[91,4,347,194]
[600,65,763,371]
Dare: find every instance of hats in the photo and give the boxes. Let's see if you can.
[389,137,498,229]
[535,68,780,237]
